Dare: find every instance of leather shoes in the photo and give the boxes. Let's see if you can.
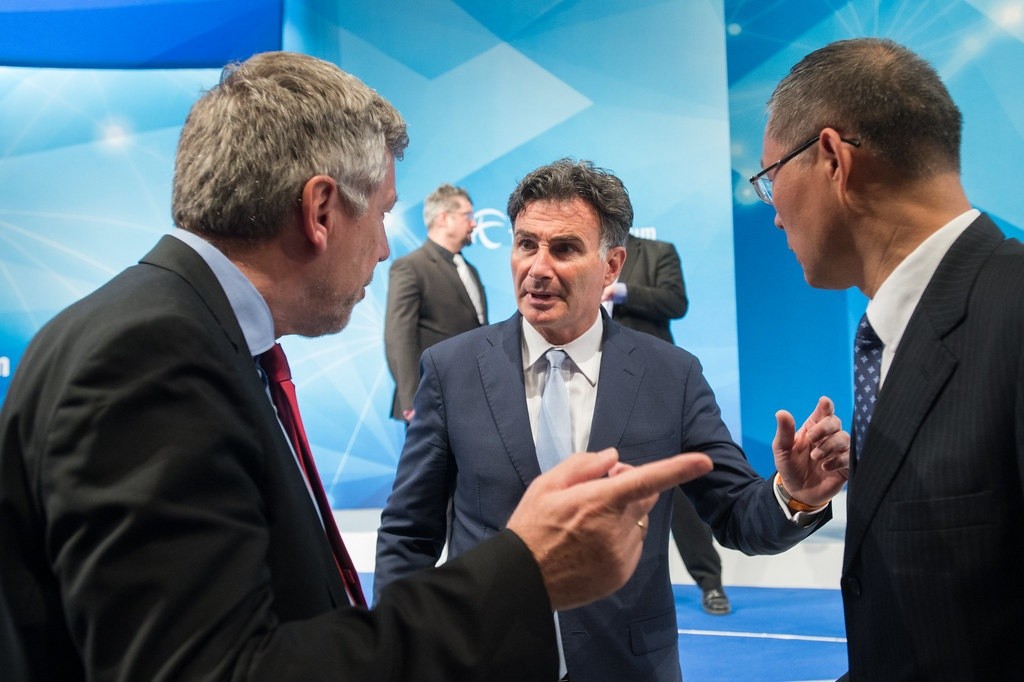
[702,586,730,614]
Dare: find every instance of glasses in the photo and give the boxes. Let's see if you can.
[749,133,861,205]
[444,210,476,221]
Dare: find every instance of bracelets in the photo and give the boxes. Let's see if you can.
[777,476,829,512]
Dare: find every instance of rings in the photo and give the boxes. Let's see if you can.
[637,521,647,540]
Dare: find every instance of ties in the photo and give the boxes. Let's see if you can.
[852,312,885,462]
[535,349,574,474]
[257,340,368,609]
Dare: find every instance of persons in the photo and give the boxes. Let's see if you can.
[373,157,851,681]
[761,37,1023,682]
[384,185,488,431]
[0,52,712,682]
[600,234,731,614]
